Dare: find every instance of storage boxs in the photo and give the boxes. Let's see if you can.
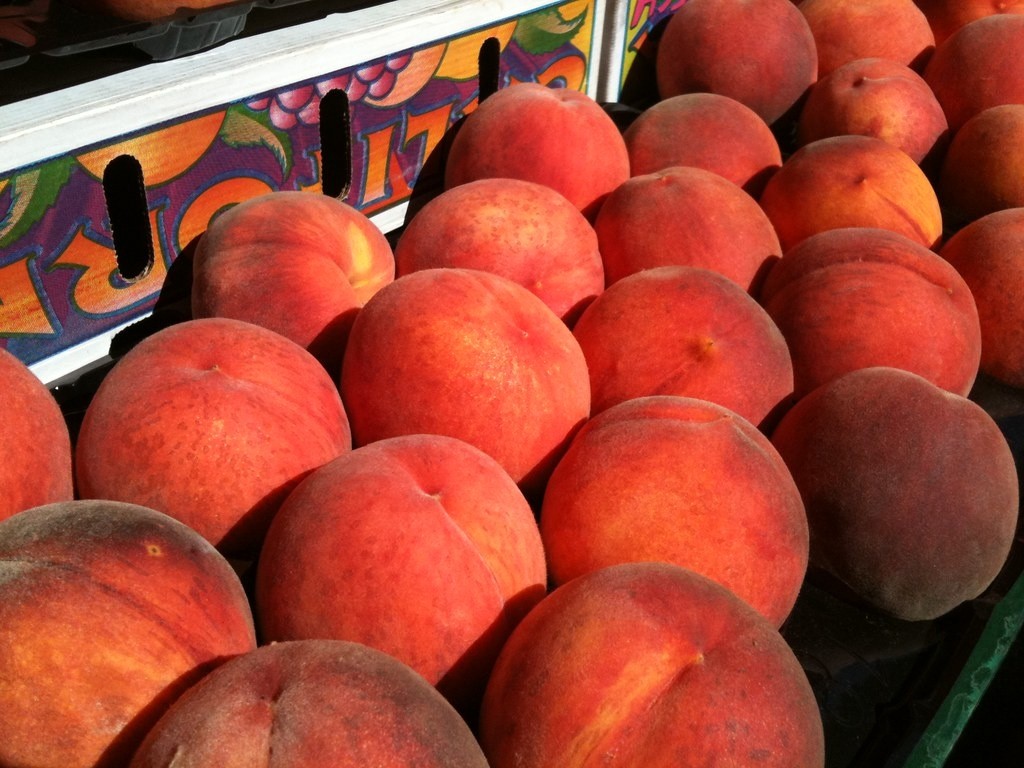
[0,0,1024,768]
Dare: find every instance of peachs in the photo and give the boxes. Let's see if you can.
[0,0,1024,768]
